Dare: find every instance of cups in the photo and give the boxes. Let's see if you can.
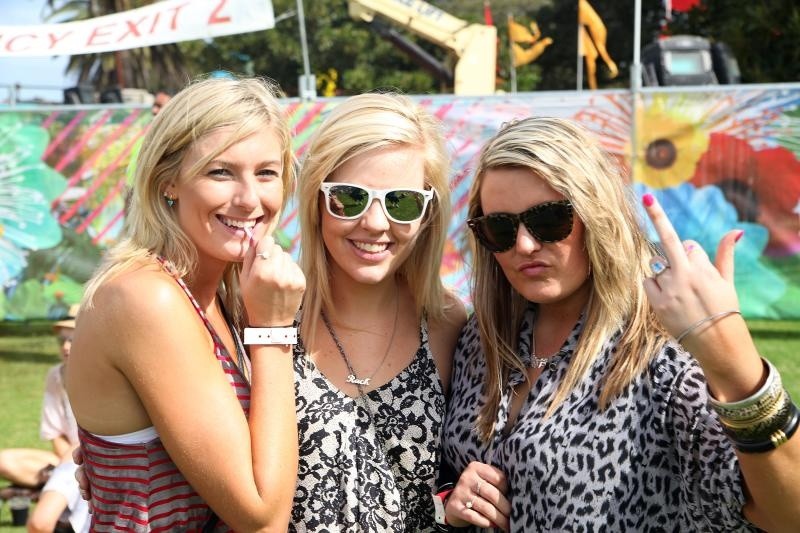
[121,88,146,102]
[10,497,31,524]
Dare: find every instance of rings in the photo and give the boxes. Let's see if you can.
[686,244,701,254]
[476,479,486,494]
[650,259,671,280]
[465,495,479,509]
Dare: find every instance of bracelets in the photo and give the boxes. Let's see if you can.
[676,308,742,342]
[705,356,783,420]
[243,326,298,345]
[717,386,792,441]
[723,402,800,453]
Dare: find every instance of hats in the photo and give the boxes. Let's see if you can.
[53,304,82,333]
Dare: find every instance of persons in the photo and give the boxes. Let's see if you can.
[71,91,469,533]
[64,70,307,533]
[431,114,800,533]
[24,303,92,533]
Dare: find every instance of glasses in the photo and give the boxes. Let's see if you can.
[320,181,435,225]
[57,335,75,346]
[467,197,573,253]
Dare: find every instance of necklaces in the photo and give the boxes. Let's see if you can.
[320,274,400,386]
[528,320,560,369]
